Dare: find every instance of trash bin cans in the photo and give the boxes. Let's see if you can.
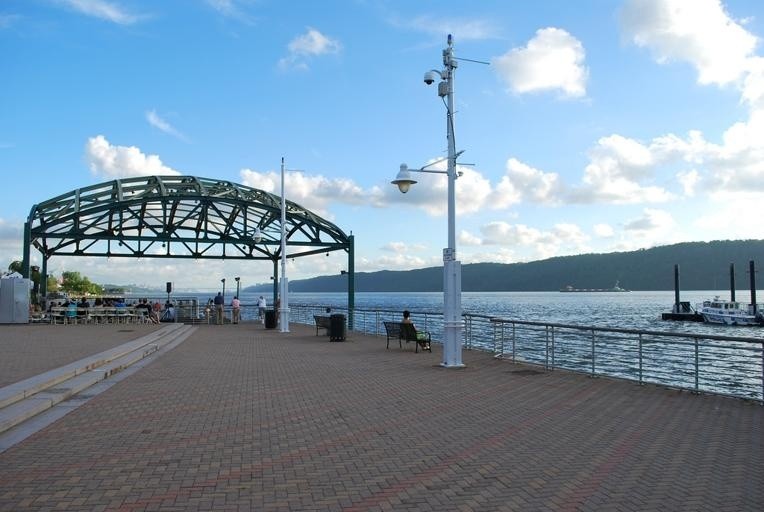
[265,309,278,329]
[329,313,345,341]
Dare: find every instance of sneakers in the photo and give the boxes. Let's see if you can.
[422,346,429,350]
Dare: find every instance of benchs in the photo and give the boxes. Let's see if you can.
[381,322,431,354]
[312,316,331,336]
[49,307,151,325]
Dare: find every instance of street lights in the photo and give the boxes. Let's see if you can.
[251,227,291,333]
[392,149,464,367]
[235,277,240,299]
[221,279,225,296]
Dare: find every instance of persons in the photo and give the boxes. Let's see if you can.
[232,296,240,324]
[210,299,214,311]
[208,298,211,305]
[214,292,224,325]
[400,310,430,350]
[275,294,280,322]
[29,296,174,324]
[323,308,332,318]
[257,296,266,324]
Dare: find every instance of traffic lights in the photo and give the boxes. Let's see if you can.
[167,283,171,292]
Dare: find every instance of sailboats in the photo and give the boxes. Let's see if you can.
[661,258,764,325]
